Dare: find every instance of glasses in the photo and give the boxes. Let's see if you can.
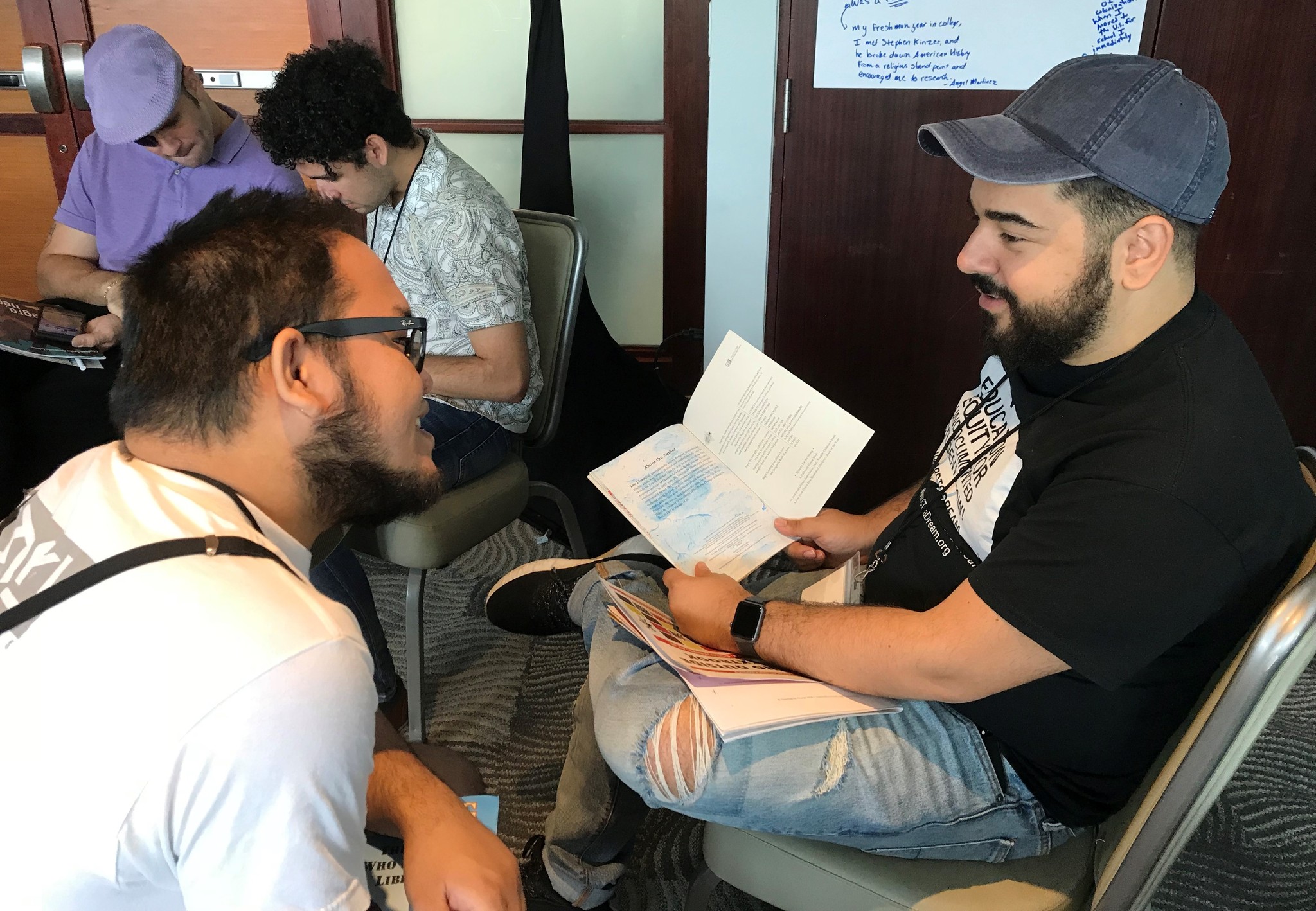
[241,316,427,374]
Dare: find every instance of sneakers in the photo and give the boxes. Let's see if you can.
[510,829,618,911]
[484,533,681,637]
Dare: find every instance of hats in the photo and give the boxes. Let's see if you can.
[81,22,183,147]
[917,52,1231,225]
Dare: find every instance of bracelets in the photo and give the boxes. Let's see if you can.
[104,275,129,305]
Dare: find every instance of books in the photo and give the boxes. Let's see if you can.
[0,293,107,368]
[596,574,904,743]
[587,329,875,583]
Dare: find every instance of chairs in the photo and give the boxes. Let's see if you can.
[698,440,1316,911]
[341,210,591,744]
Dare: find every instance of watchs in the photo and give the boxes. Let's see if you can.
[730,596,773,664]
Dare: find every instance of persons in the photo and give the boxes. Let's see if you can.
[0,185,525,911]
[485,53,1316,911]
[36,24,307,348]
[248,38,544,733]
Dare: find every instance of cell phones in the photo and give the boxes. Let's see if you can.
[32,307,86,342]
